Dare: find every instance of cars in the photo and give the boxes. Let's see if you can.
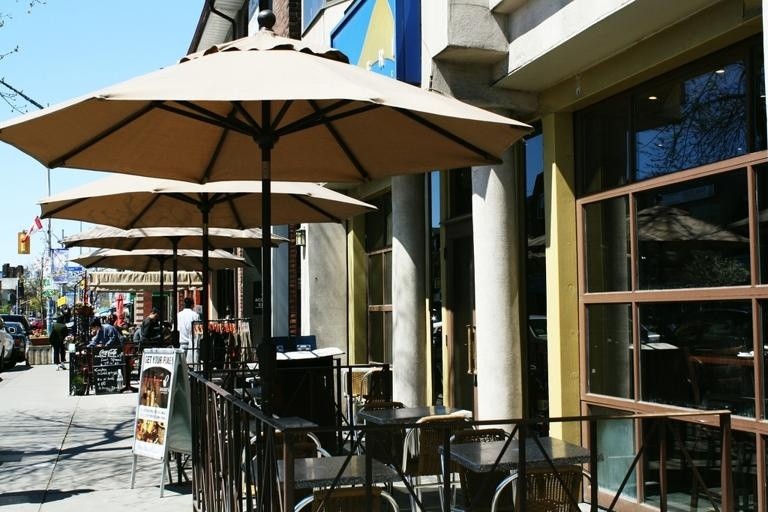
[0,314,32,370]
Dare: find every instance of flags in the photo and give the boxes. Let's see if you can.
[19,217,44,244]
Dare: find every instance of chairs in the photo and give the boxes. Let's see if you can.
[209,369,605,512]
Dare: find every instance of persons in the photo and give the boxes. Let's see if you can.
[51,297,201,395]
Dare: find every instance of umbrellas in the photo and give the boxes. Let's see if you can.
[0,10,534,511]
[69,249,252,327]
[34,172,380,375]
[55,225,292,348]
[527,200,768,260]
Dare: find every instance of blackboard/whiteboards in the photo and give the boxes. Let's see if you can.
[92,344,128,395]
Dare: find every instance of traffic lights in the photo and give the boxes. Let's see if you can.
[2,263,9,278]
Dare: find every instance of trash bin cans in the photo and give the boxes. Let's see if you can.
[258,335,346,456]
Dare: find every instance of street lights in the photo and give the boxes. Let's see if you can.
[18,217,51,335]
